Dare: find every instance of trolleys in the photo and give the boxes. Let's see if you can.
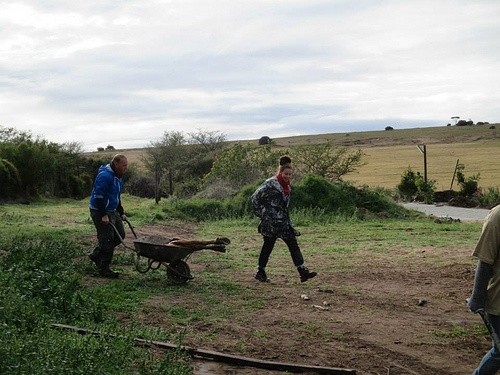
[105,218,226,287]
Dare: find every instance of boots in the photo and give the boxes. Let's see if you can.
[298,267,317,283]
[256,269,270,282]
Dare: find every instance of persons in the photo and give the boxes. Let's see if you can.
[466,204,500,374]
[87,153,128,278]
[251,155,318,283]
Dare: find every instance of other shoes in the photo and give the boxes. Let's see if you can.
[89,248,100,263]
[100,268,117,277]
